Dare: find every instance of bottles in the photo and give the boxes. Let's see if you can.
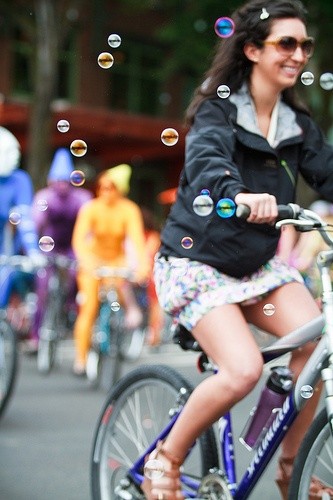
[245,366,293,446]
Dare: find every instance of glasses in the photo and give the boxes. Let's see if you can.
[254,36,314,57]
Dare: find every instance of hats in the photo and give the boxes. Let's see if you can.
[48,148,81,182]
[105,164,133,193]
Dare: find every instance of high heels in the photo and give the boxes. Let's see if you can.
[272,455,333,500]
[141,438,186,500]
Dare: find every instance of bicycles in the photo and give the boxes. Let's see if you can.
[90,200,333,500]
[1,253,136,421]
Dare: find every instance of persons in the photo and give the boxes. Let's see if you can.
[140,0,333,500]
[0,125,150,378]
